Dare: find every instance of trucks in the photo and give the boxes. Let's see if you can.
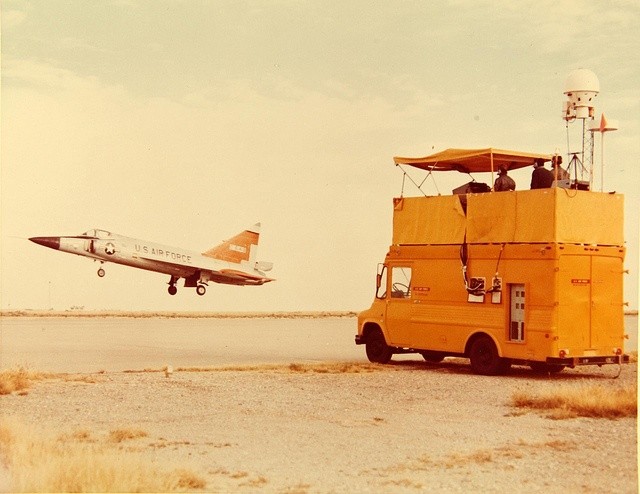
[355,244,629,374]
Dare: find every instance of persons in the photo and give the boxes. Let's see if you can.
[494,163,515,191]
[551,155,569,180]
[530,157,552,189]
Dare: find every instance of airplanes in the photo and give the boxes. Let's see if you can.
[29,221,276,295]
